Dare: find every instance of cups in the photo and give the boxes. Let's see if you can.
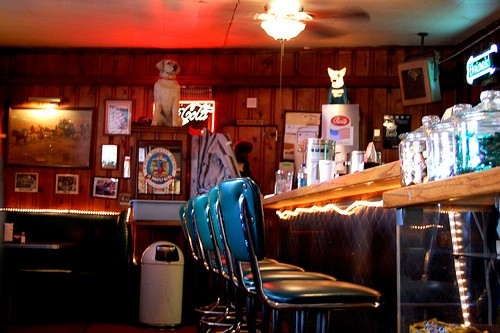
[319,160,335,183]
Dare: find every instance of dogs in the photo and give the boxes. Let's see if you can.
[326,67,348,104]
[150,58,183,127]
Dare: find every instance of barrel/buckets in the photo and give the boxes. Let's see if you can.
[303,138,335,187]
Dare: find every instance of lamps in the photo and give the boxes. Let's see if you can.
[254,0,312,98]
[466,44,497,85]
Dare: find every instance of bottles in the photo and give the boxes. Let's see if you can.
[275,169,293,195]
[398,89,500,185]
[297,164,307,188]
[21,232,25,244]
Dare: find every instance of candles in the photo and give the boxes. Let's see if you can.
[4,223,13,241]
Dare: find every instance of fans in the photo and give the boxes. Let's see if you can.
[218,0,370,39]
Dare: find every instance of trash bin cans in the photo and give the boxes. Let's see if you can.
[138,241,185,328]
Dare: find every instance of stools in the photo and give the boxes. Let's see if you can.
[179,176,384,333]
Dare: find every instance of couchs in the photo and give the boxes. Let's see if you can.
[0,209,129,323]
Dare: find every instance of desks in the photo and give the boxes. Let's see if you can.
[382,166,500,333]
[263,160,400,333]
[0,242,89,333]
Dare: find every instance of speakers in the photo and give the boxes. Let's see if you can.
[398,57,440,106]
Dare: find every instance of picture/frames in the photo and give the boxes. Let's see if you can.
[91,176,120,200]
[103,99,133,136]
[5,106,96,169]
[100,144,119,170]
[54,173,81,195]
[15,173,38,192]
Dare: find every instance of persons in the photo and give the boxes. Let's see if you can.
[234,140,262,195]
[99,182,110,194]
